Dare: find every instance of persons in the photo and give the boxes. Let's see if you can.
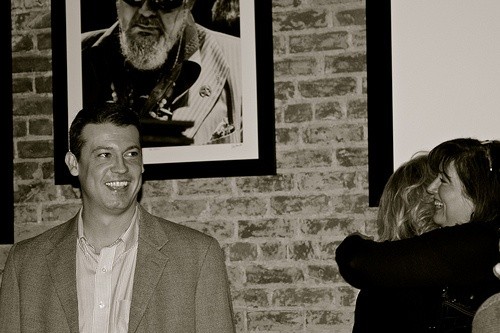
[335,137,500,333]
[80,0,243,142]
[1,106,236,333]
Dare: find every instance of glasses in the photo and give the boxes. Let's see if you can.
[480,139,492,171]
[123,0,184,14]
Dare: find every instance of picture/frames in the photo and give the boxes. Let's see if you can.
[50,0,278,186]
